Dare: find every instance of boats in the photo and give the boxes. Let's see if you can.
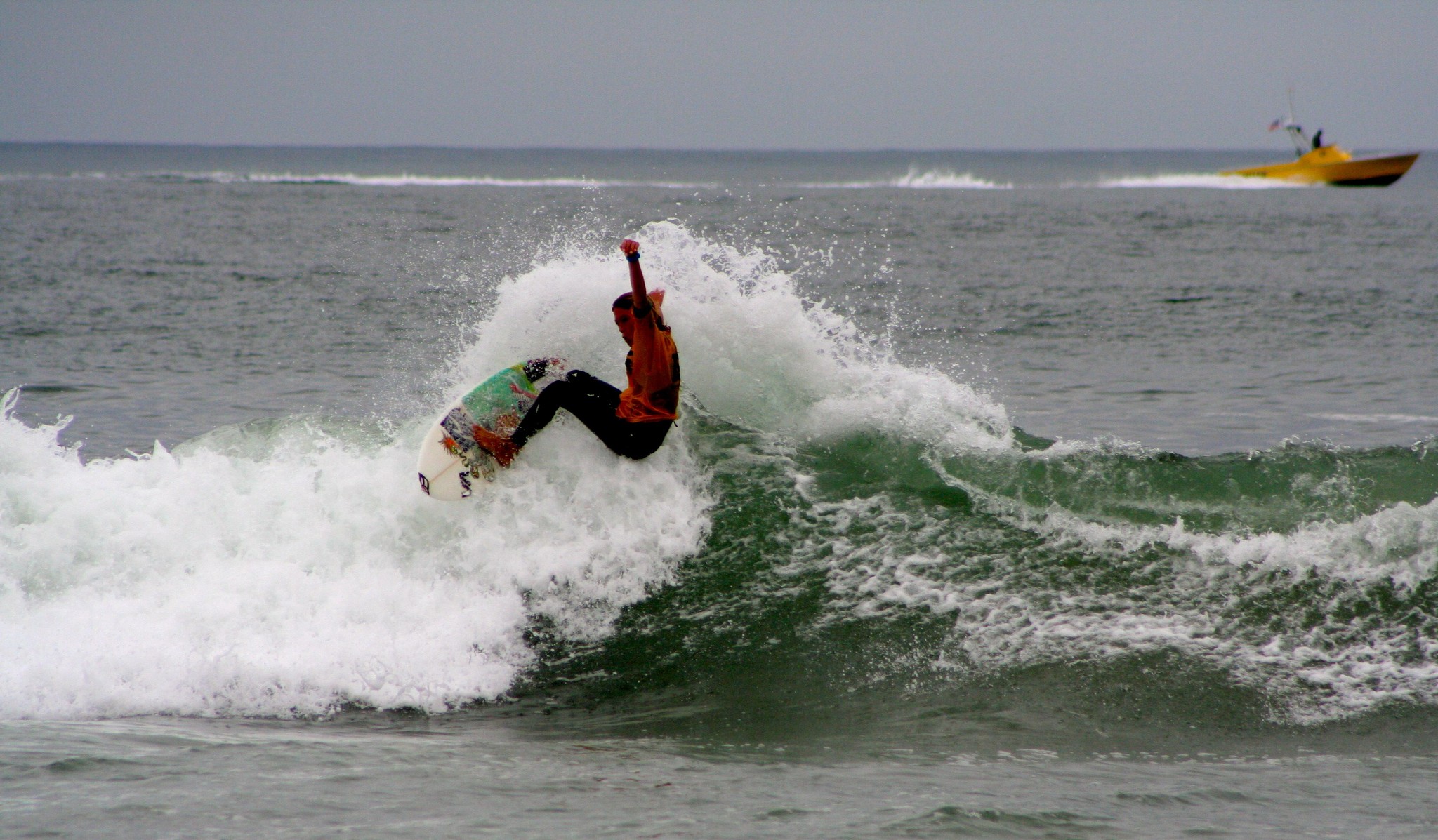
[1216,87,1420,186]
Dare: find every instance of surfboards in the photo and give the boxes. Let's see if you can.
[417,357,565,503]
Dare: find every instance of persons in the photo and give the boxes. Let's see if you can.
[472,239,680,467]
[1311,129,1322,150]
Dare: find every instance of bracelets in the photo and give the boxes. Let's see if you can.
[626,253,640,262]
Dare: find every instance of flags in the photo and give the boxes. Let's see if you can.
[1270,120,1279,130]
